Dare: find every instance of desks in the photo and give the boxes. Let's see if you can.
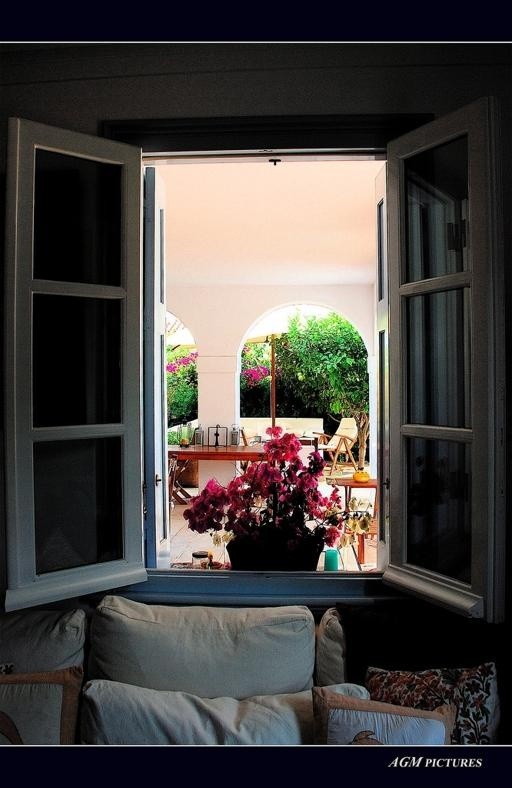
[336,477,377,518]
[168,444,273,504]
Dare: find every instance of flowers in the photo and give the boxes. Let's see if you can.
[183,427,369,547]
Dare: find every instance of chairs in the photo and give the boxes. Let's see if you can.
[313,418,359,476]
[170,456,191,505]
[168,454,178,503]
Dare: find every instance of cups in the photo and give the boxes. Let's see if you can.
[323,549,339,571]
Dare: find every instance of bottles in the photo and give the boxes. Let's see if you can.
[352,470,370,484]
[177,418,204,448]
[230,423,240,447]
[191,550,215,569]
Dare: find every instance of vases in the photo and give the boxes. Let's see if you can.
[227,536,324,570]
